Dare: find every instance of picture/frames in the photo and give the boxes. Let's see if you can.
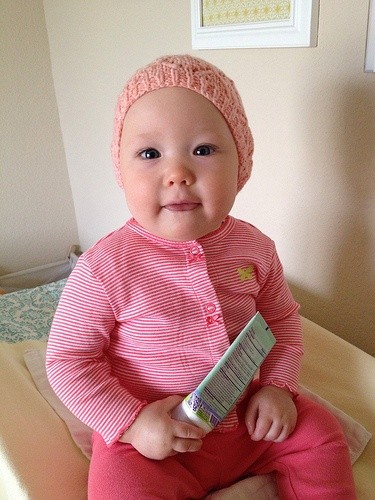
[190,0,319,50]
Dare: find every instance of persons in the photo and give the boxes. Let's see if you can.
[44,53,356,500]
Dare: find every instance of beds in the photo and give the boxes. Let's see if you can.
[0,245,375,500]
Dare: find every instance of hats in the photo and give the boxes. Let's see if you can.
[110,54,253,190]
